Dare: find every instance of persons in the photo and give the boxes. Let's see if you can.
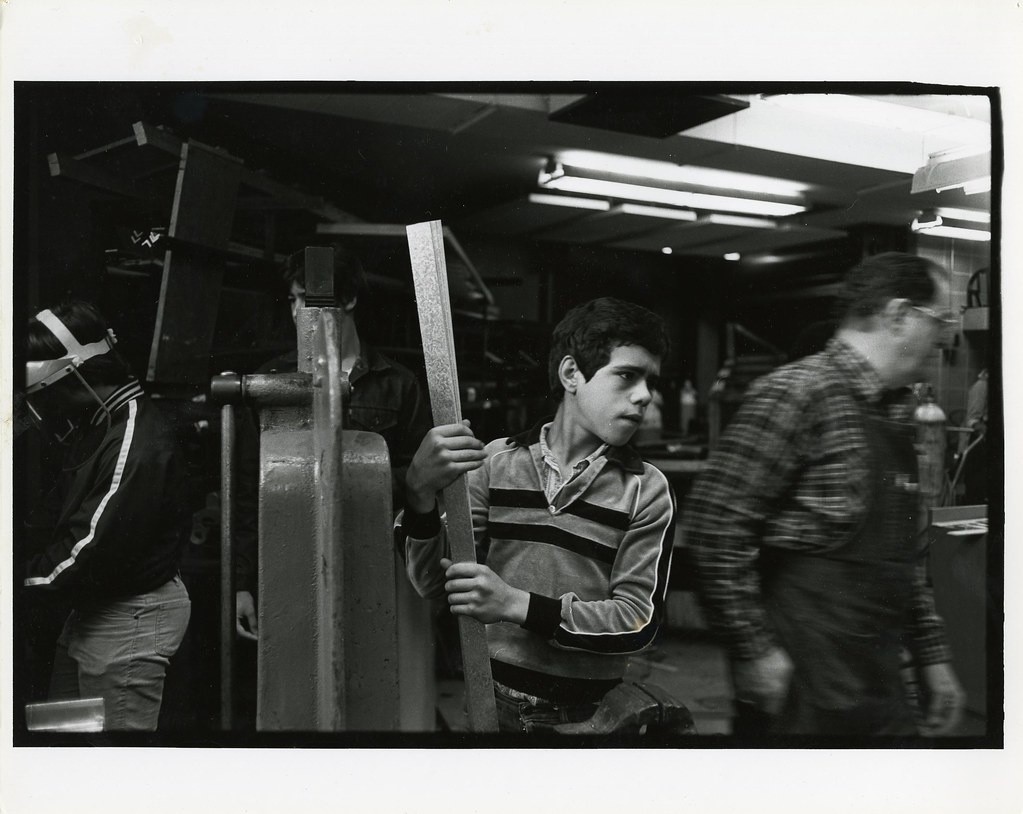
[682,251,964,740]
[234,244,434,640]
[958,380,988,455]
[394,298,678,731]
[22,299,191,732]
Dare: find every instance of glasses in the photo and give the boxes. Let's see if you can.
[911,305,958,340]
